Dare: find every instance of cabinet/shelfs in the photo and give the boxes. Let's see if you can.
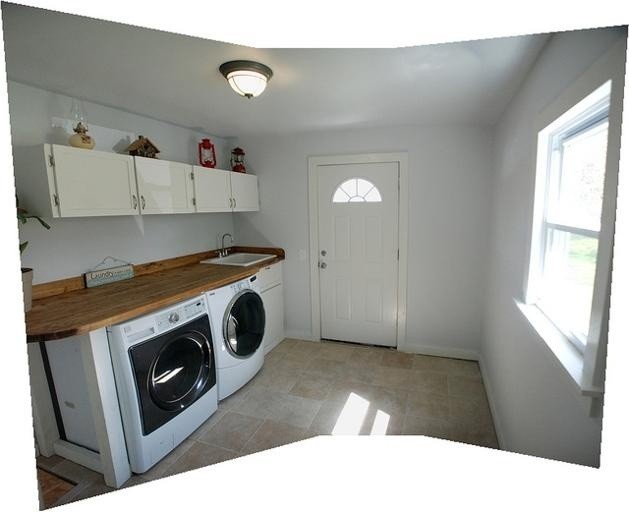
[16,142,196,219]
[193,165,259,213]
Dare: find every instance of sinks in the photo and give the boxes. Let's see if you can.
[199,250,278,267]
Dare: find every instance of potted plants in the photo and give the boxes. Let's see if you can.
[17,207,50,312]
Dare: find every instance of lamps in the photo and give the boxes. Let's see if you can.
[198,138,217,168]
[70,95,95,149]
[230,146,246,174]
[218,60,273,99]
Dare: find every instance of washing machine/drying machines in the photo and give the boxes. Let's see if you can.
[106,295,219,475]
[206,275,267,402]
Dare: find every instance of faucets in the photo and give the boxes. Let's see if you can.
[221,232,233,255]
